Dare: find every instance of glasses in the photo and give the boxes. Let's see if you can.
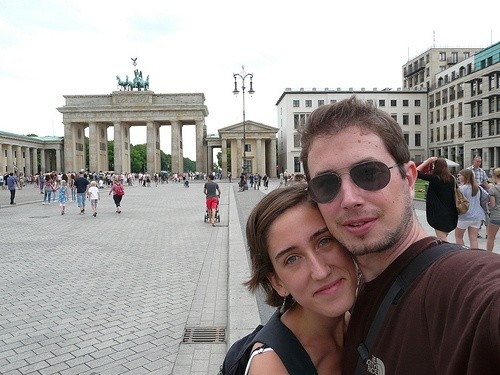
[309,161,400,203]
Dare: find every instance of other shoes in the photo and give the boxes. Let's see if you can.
[212,222,215,227]
[93,212,97,217]
[48,203,51,205]
[10,202,16,205]
[81,210,85,215]
[41,192,44,194]
[43,203,45,205]
[62,211,64,215]
[116,210,121,213]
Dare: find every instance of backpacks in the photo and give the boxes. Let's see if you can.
[114,183,125,195]
[222,306,318,375]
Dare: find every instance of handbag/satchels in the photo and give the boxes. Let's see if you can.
[147,179,150,182]
[452,176,470,213]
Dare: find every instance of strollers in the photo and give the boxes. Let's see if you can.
[203,195,221,223]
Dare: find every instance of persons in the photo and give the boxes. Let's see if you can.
[107,177,126,215]
[486,167,500,252]
[277,168,305,187]
[217,183,360,374]
[28,167,153,205]
[203,174,221,227]
[466,155,490,239]
[53,179,70,215]
[155,170,201,187]
[86,180,100,217]
[73,171,90,215]
[236,171,269,192]
[8,173,17,205]
[455,168,491,249]
[415,156,459,242]
[0,172,16,190]
[299,97,500,375]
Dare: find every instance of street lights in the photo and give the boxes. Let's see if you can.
[232,64,256,190]
